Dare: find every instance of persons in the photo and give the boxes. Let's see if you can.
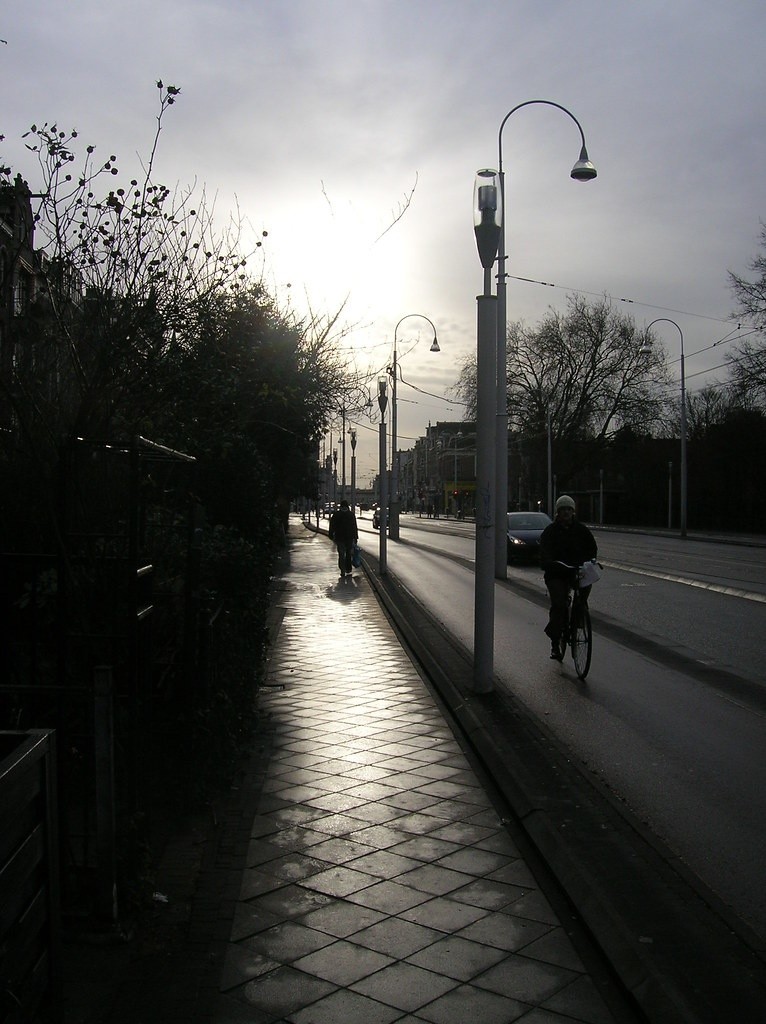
[427,503,433,518]
[329,500,358,577]
[540,495,598,660]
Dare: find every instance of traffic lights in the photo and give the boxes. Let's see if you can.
[453,490,458,495]
[417,492,423,498]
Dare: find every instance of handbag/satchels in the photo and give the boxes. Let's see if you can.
[351,547,361,568]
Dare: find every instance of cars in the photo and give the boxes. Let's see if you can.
[507,512,553,565]
[324,504,335,514]
[373,508,391,529]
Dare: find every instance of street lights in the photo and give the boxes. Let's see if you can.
[458,419,477,513]
[334,451,337,513]
[436,430,457,518]
[639,319,687,537]
[521,388,552,519]
[378,382,389,576]
[352,432,357,513]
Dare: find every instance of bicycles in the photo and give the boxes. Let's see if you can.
[549,556,602,679]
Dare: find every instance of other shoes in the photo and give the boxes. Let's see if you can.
[551,640,561,657]
[345,567,352,573]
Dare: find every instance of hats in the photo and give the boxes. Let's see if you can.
[556,495,575,510]
[341,501,348,506]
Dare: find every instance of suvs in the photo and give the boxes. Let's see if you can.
[361,504,369,511]
